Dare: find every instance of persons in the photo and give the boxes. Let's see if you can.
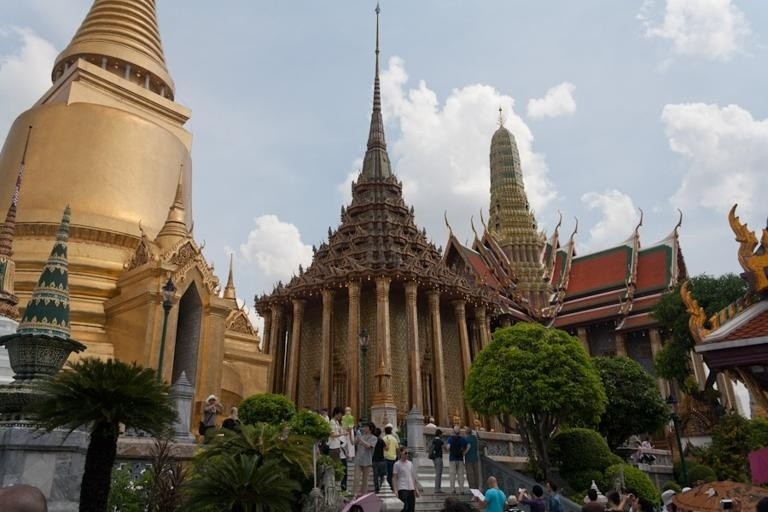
[635,437,656,465]
[199,395,224,444]
[476,476,692,512]
[221,407,243,432]
[314,404,480,512]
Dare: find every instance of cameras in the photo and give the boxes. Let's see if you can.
[720,499,734,510]
[519,488,526,494]
[628,494,635,500]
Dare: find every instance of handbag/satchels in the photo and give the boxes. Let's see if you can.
[199,421,206,436]
[429,444,438,459]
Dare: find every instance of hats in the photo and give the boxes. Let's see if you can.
[661,490,677,506]
[507,495,518,505]
[206,395,218,404]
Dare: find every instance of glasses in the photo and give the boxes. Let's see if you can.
[402,452,410,455]
